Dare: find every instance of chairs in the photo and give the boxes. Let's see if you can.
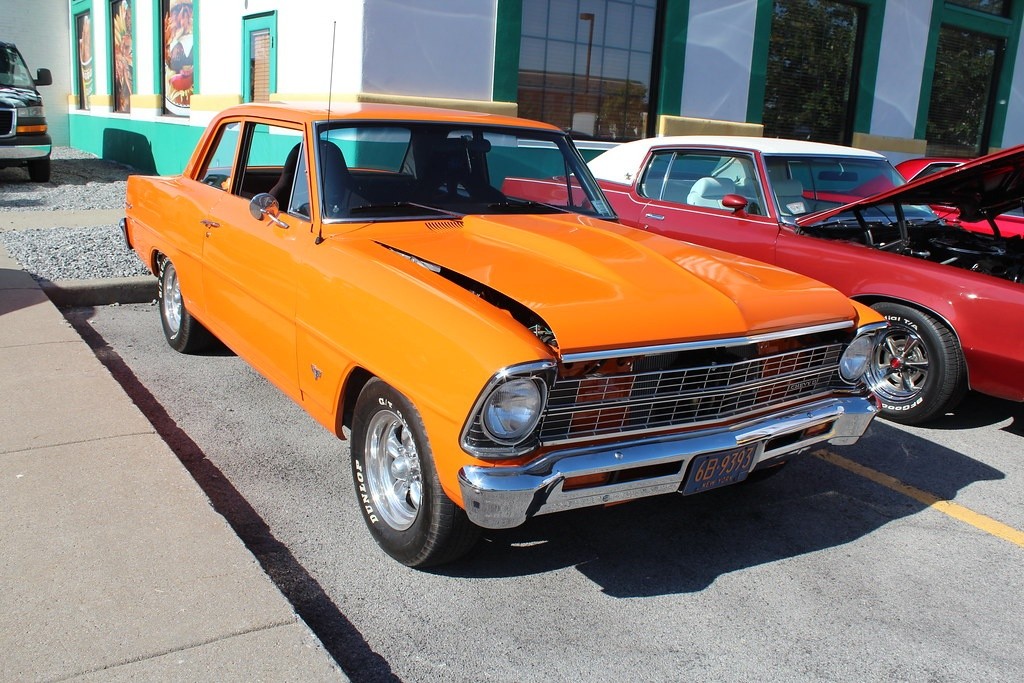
[685,177,737,213]
[263,140,374,221]
[770,175,812,216]
[398,139,508,213]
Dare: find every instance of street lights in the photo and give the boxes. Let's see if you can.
[579,13,595,110]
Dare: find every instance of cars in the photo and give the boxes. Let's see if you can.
[805,156,1024,238]
[503,134,1024,426]
[119,91,899,570]
[0,41,52,182]
